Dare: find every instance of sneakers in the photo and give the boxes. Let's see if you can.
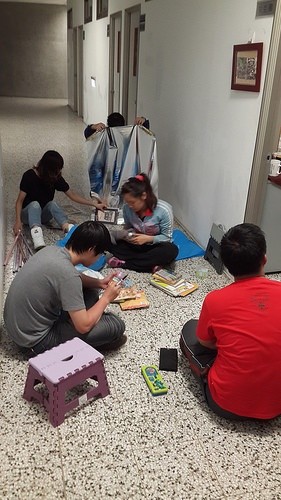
[62,222,74,233]
[30,227,45,249]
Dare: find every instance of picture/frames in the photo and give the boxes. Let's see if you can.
[95,208,119,225]
[231,42,263,92]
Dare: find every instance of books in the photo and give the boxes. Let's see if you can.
[105,269,199,311]
[95,208,120,225]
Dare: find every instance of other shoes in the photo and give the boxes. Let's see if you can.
[107,257,126,269]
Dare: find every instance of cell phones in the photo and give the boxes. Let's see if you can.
[108,270,128,286]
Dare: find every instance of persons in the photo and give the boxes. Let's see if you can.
[180,222,281,422]
[3,221,128,356]
[13,112,183,278]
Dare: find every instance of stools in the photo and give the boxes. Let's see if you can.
[22,337,111,428]
[204,222,226,275]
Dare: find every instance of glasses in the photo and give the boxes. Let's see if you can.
[100,251,107,256]
